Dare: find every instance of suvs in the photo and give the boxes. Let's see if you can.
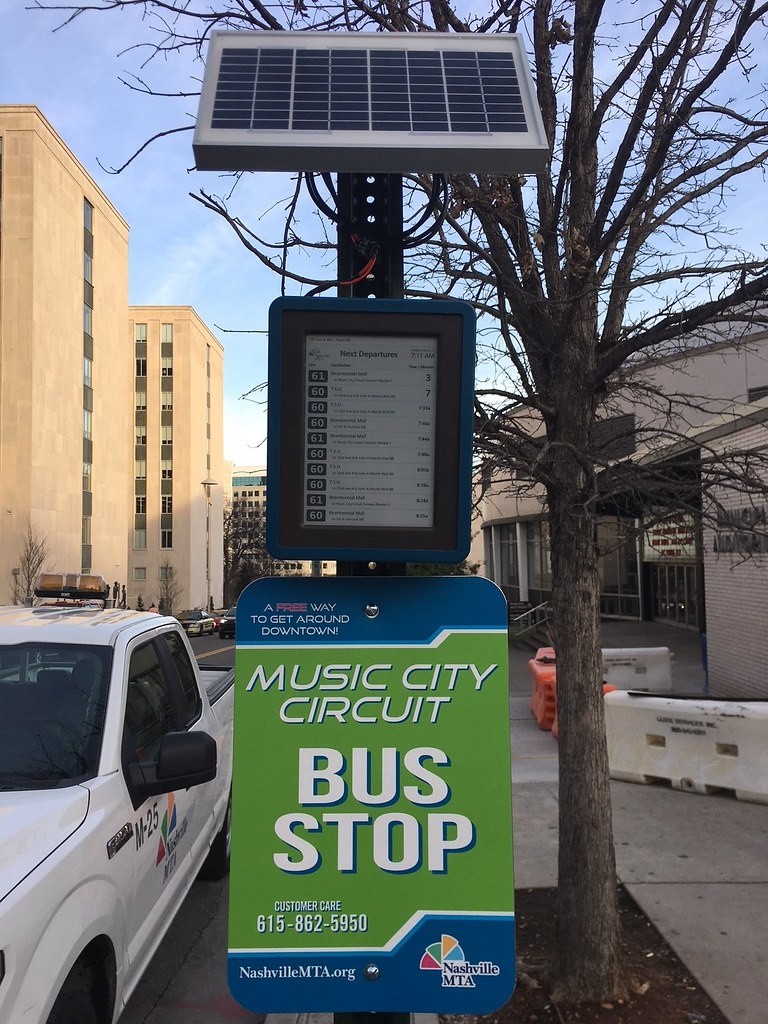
[218,605,237,639]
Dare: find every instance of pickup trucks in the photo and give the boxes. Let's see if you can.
[1,570,239,1022]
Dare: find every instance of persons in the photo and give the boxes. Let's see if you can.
[148,602,160,613]
[107,581,126,609]
[135,603,145,611]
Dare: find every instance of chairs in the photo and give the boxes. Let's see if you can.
[0,657,103,736]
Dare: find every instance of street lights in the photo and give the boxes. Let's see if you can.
[201,478,218,613]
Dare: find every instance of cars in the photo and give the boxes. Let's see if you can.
[175,609,216,637]
[210,610,229,632]
